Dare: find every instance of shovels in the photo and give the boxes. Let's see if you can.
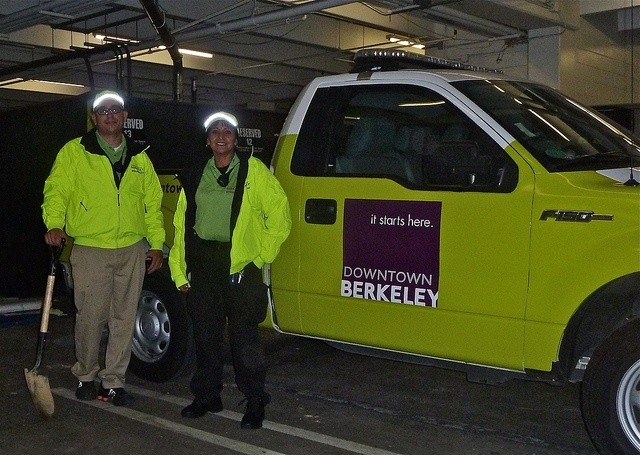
[24,237,65,422]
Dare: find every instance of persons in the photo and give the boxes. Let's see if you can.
[168,111,295,430]
[40,90,166,407]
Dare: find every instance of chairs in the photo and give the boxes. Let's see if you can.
[328,116,417,184]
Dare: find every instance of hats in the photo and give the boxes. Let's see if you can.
[204,111,238,132]
[93,90,125,111]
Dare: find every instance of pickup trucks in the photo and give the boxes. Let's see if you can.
[111,48,640,455]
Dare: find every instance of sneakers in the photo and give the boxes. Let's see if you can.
[238,397,265,428]
[181,394,223,417]
[77,380,96,400]
[97,380,136,406]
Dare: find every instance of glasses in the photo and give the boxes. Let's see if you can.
[94,106,124,114]
